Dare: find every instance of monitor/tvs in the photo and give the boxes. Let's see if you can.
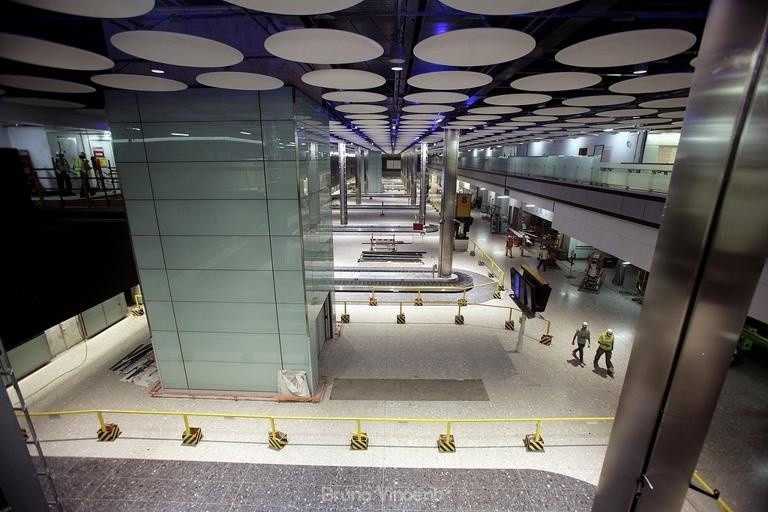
[579,148,587,155]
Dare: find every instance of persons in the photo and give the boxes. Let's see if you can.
[55,150,96,196]
[572,322,591,363]
[594,329,615,374]
[536,245,550,272]
[520,234,528,255]
[505,235,513,258]
